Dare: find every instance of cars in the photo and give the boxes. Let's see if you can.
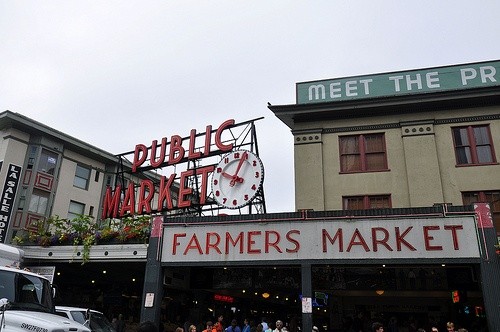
[0,266,115,332]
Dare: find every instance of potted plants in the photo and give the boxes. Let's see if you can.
[10,215,153,265]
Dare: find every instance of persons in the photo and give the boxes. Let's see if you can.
[372,322,384,332]
[176,313,274,332]
[432,322,468,332]
[111,314,127,332]
[273,320,288,332]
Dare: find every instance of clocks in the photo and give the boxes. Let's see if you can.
[211,150,264,210]
